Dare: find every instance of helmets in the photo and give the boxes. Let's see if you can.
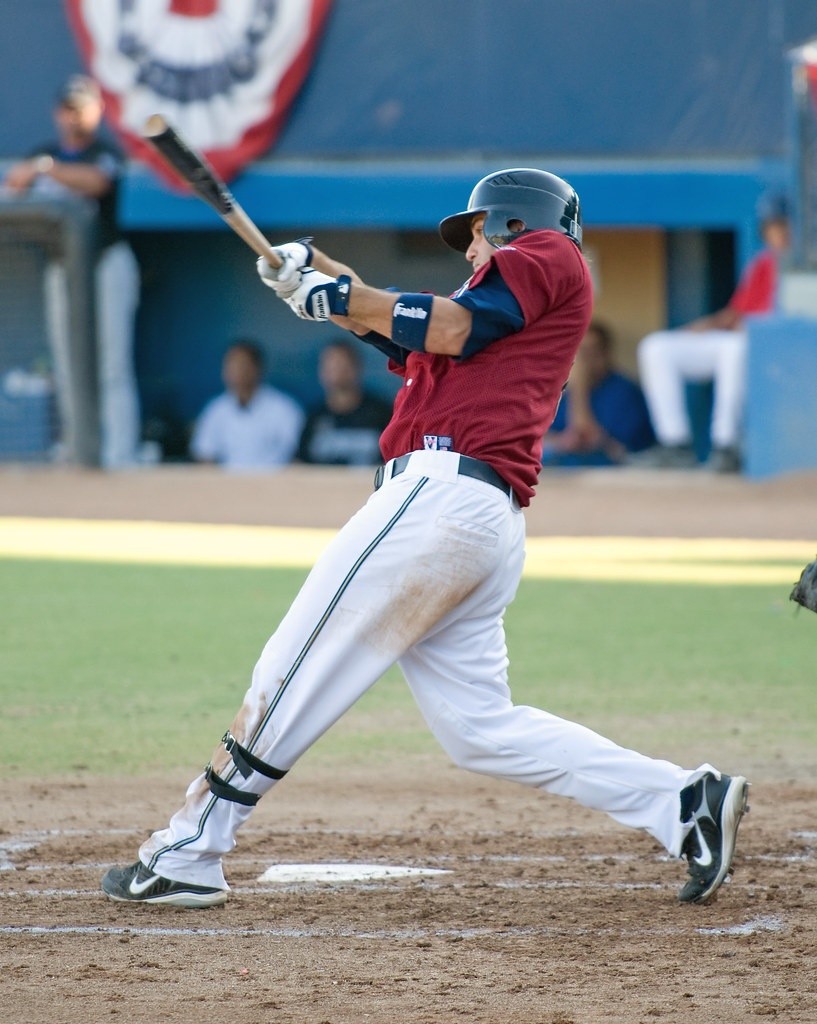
[439,167,582,251]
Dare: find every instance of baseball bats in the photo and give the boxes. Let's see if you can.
[144,113,285,269]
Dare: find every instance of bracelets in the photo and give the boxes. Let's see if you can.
[40,155,52,171]
[391,292,435,353]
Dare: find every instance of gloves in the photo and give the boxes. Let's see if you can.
[256,236,316,299]
[283,266,353,322]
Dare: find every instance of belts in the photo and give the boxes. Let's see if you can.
[374,453,511,499]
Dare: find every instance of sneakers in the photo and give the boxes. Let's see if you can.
[676,771,750,904]
[102,860,227,908]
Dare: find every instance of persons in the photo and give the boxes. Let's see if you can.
[541,324,657,466]
[8,74,141,463]
[103,167,748,907]
[639,193,793,471]
[294,341,392,464]
[188,337,307,465]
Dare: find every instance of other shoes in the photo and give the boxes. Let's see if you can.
[704,443,741,473]
[629,439,697,466]
